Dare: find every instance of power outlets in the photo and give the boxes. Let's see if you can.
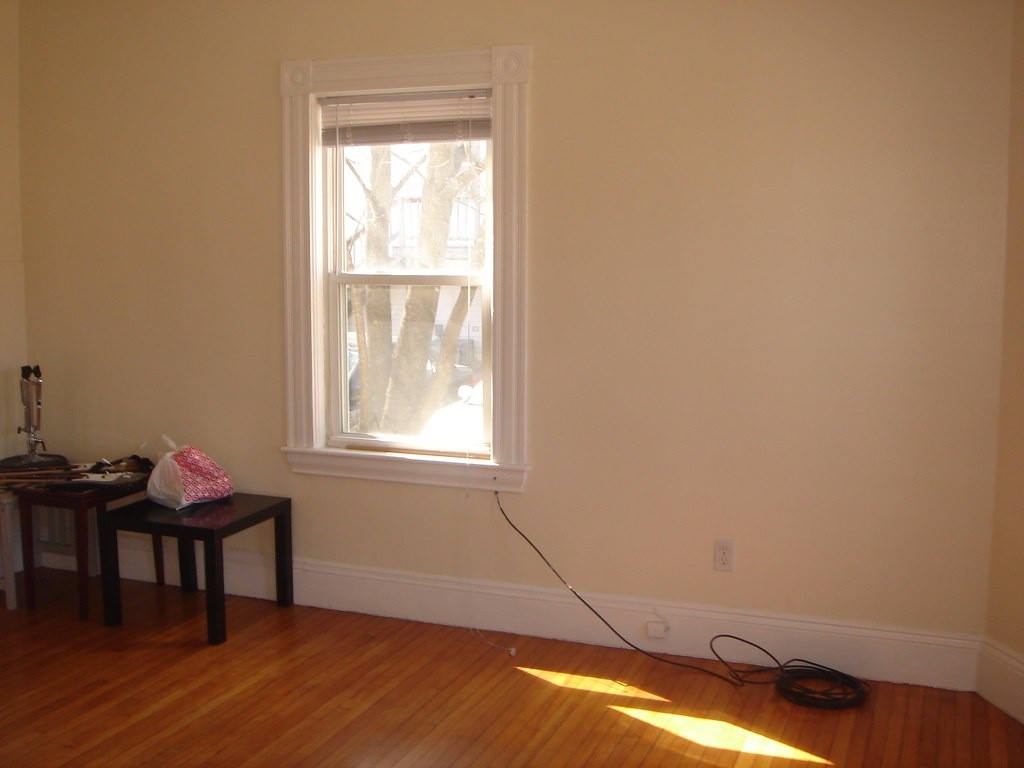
[713,538,733,571]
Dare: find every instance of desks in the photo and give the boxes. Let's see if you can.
[12,472,164,620]
[99,492,292,645]
[0,464,98,608]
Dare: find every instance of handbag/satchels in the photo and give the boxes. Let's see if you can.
[145,434,233,511]
